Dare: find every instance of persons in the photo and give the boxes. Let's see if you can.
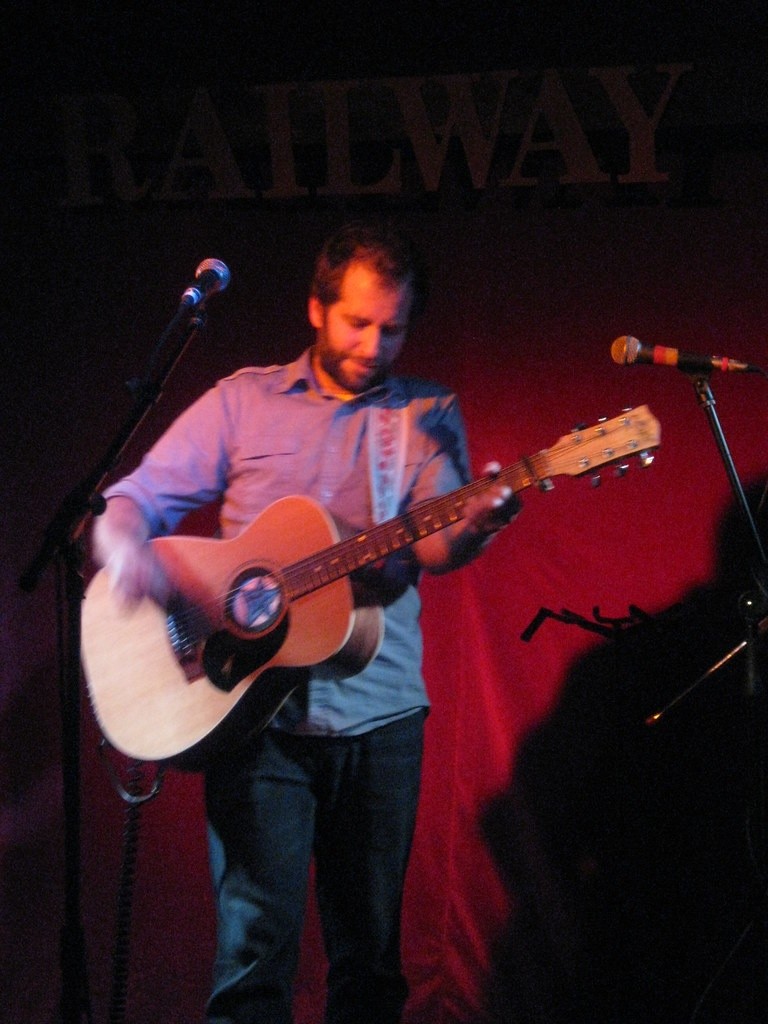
[89,220,521,1024]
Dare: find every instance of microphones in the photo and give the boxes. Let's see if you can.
[180,258,230,309]
[611,335,761,373]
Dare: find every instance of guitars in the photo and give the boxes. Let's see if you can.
[78,400,664,775]
